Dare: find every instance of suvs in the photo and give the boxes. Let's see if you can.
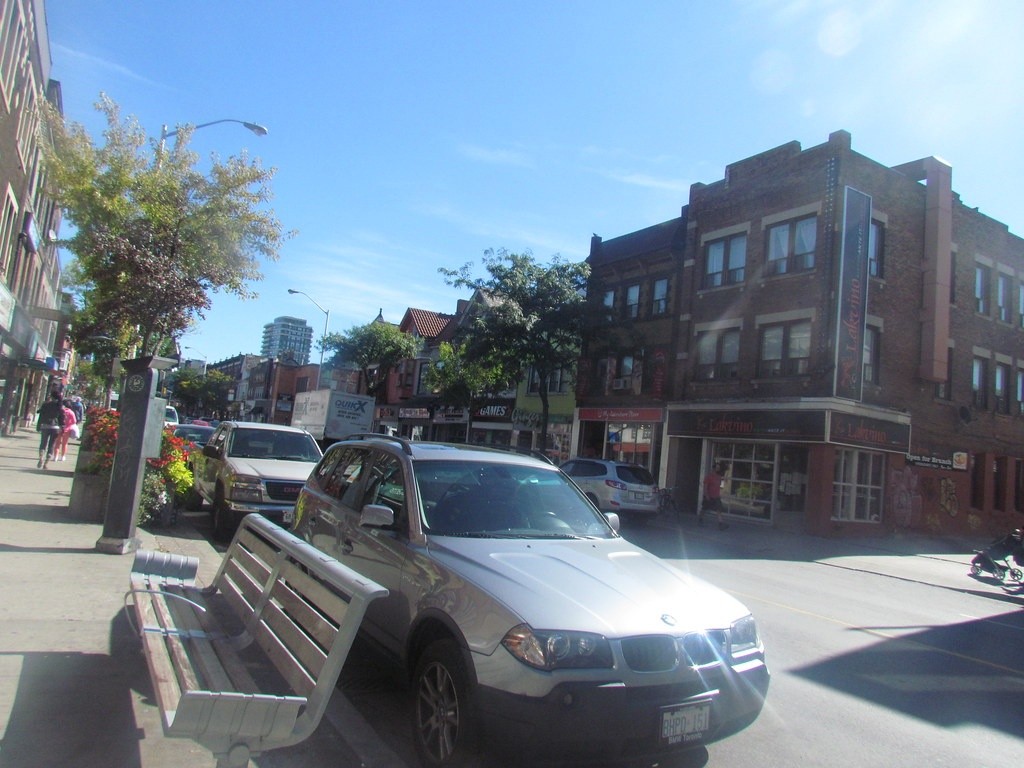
[165,406,180,432]
[280,430,773,768]
[559,458,659,526]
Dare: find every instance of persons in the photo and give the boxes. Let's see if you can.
[36,395,51,431]
[36,391,66,469]
[53,400,77,462]
[71,397,83,425]
[697,463,729,531]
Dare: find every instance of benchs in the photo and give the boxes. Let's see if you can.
[128,513,389,768]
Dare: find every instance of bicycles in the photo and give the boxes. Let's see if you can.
[656,485,681,524]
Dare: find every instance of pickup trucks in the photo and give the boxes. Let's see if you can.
[191,421,326,540]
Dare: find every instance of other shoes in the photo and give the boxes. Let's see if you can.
[37,460,43,468]
[55,458,58,461]
[61,457,65,461]
[43,464,48,469]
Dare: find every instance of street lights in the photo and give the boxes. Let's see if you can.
[287,288,331,391]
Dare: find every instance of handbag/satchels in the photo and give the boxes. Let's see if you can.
[70,424,79,439]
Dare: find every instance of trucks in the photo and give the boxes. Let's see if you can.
[288,389,375,458]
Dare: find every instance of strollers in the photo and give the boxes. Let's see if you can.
[971,528,1023,582]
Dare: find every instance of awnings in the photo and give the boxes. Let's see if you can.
[36,356,59,375]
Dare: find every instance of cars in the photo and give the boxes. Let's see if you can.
[169,423,220,451]
[182,412,220,429]
